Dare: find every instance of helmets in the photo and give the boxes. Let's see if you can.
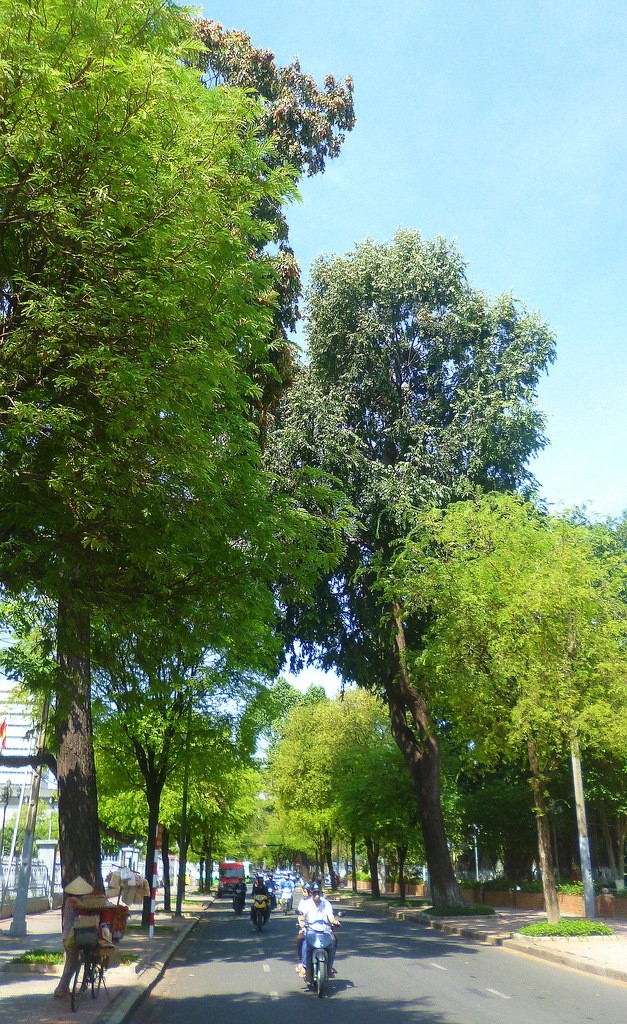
[309,883,320,892]
[303,882,311,890]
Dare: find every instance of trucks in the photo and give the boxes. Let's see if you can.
[215,862,250,899]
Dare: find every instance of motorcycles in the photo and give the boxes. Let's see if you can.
[268,887,278,913]
[232,888,248,915]
[294,909,346,999]
[280,888,295,916]
[251,892,273,932]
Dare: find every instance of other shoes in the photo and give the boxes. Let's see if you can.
[53,987,72,998]
[265,920,271,923]
[298,968,305,978]
[328,972,334,978]
[331,969,337,974]
[295,964,301,971]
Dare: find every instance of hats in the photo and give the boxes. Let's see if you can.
[64,876,93,895]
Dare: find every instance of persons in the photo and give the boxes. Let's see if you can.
[250,872,295,908]
[296,881,340,978]
[232,877,247,908]
[54,876,94,997]
[250,878,271,923]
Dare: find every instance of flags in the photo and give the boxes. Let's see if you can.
[0,719,7,748]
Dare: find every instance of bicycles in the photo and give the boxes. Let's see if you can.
[68,922,110,1014]
